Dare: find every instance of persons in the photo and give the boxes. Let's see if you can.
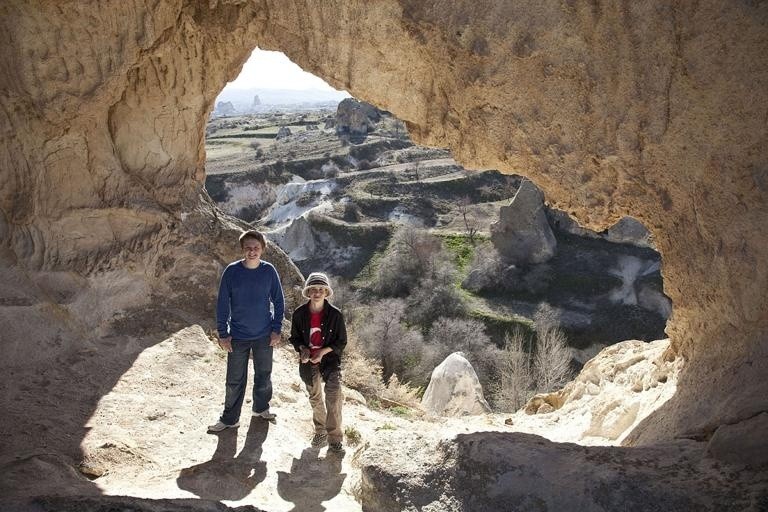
[289,271,348,452]
[207,229,285,432]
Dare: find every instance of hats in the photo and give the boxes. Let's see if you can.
[302,272,333,299]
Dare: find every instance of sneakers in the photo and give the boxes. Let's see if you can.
[329,442,343,451]
[312,433,327,445]
[208,421,240,431]
[252,409,276,419]
